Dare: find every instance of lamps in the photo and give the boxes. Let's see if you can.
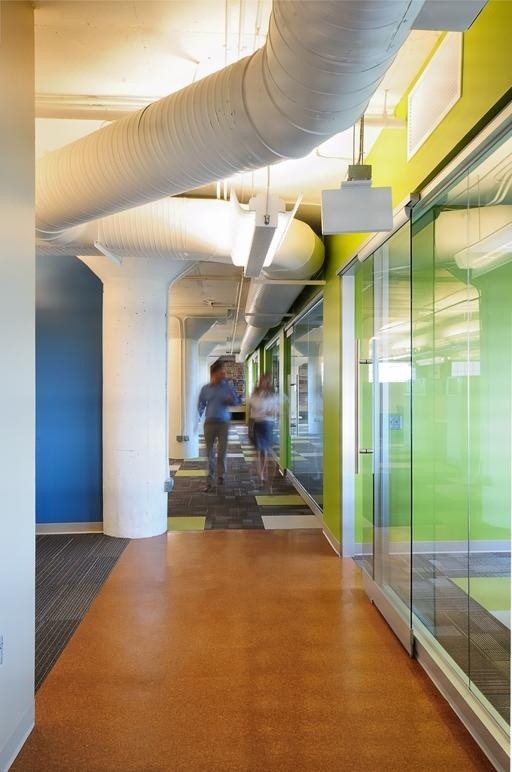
[231,166,280,282]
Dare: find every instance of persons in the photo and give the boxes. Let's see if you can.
[192,358,242,494]
[246,371,284,491]
